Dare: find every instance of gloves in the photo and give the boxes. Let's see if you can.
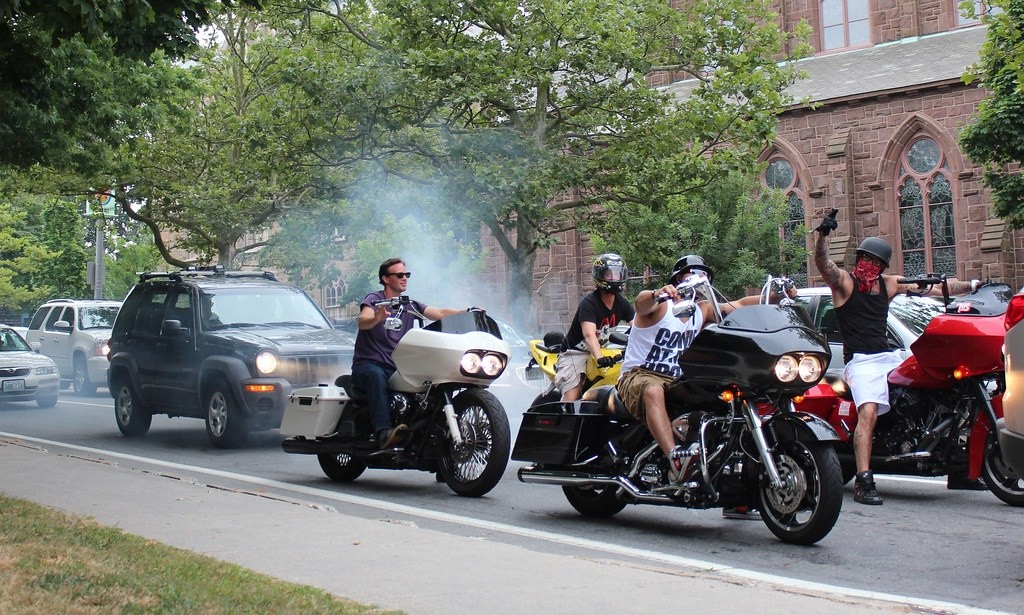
[810,208,839,236]
[597,356,616,368]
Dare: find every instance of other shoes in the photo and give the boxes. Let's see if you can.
[380,424,410,451]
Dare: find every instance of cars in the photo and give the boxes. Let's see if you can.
[0,324,60,405]
[25,297,128,394]
[106,268,360,448]
[759,285,945,375]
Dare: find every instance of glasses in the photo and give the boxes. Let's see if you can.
[384,272,411,279]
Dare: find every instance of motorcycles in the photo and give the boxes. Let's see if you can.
[747,274,1024,504]
[273,304,513,499]
[510,275,859,546]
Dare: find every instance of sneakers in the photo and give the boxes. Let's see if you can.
[723,507,763,521]
[854,470,885,505]
[669,442,700,483]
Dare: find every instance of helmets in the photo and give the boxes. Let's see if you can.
[856,237,893,269]
[666,255,714,286]
[593,254,628,294]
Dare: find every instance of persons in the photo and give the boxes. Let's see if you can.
[556,254,636,403]
[351,259,487,451]
[815,208,989,505]
[618,255,799,521]
